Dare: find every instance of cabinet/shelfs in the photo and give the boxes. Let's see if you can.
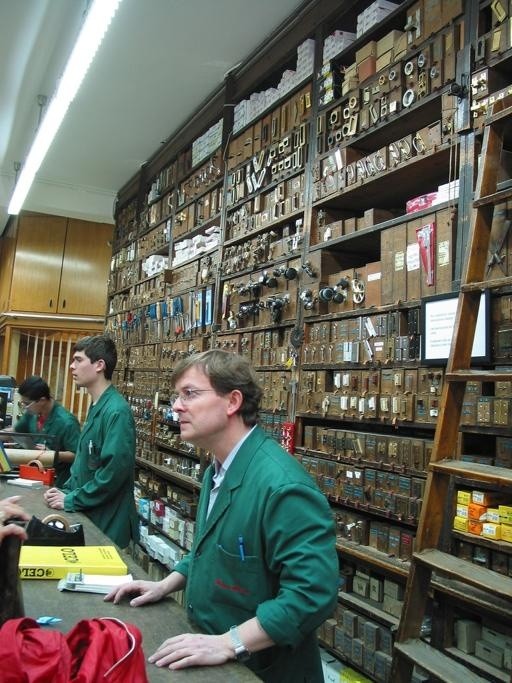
[9,213,115,317]
[90,0,512,681]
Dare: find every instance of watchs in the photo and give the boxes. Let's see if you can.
[230,625,251,664]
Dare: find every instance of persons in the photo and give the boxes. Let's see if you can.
[0,496,32,546]
[43,336,140,549]
[0,376,81,488]
[103,349,340,683]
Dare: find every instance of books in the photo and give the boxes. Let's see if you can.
[19,546,128,580]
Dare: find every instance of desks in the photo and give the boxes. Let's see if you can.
[0,477,260,682]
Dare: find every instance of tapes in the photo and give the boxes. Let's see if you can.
[42,513,70,532]
[28,459,44,473]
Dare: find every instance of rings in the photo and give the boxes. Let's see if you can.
[0,511,5,521]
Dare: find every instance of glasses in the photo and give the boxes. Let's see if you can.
[18,401,37,408]
[169,388,225,403]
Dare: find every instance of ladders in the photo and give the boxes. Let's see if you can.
[390,93,512,683]
